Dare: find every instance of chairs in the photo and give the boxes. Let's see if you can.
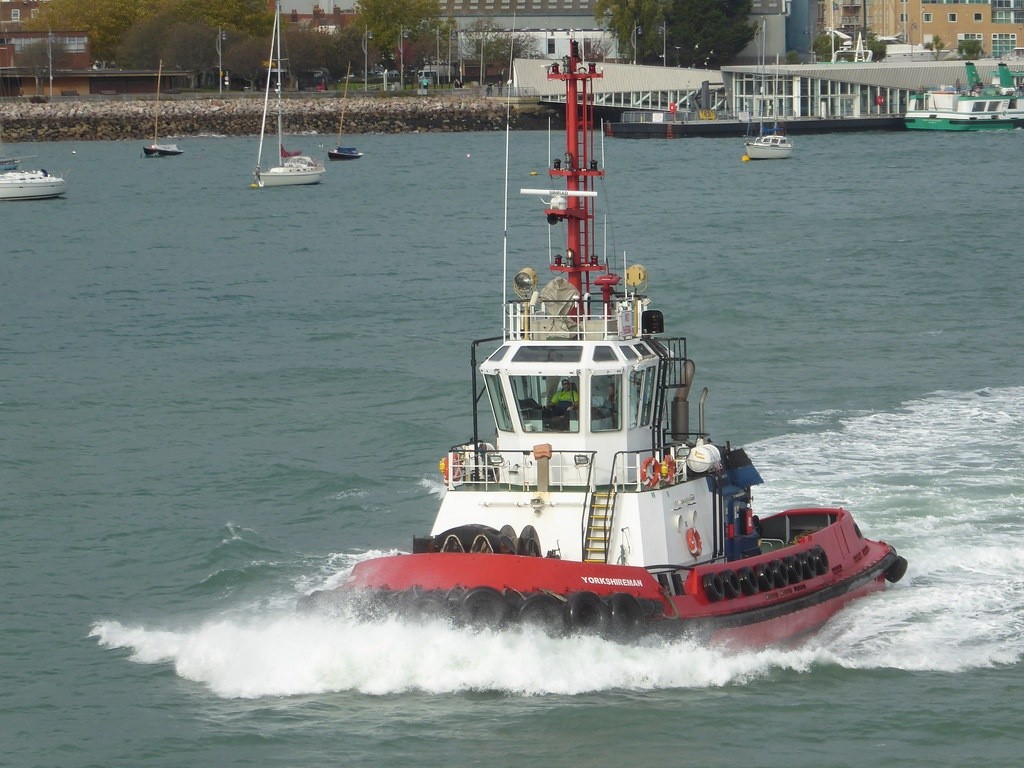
[541,382,577,426]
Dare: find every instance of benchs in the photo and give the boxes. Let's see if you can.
[519,397,554,429]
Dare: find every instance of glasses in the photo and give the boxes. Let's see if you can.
[562,383,570,385]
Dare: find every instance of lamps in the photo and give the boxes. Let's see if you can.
[531,496,543,513]
[508,464,521,476]
[491,453,503,464]
[546,213,559,226]
[513,268,538,340]
[575,455,589,465]
[626,265,647,336]
[674,443,690,458]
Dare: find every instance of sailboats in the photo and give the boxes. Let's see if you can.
[743,16,794,160]
[253,0,326,186]
[141,56,186,157]
[328,61,363,160]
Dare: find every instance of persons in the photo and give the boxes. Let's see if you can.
[543,378,579,417]
[391,82,397,91]
[418,77,429,89]
[956,79,961,94]
[592,382,614,420]
[485,87,491,96]
[498,80,503,96]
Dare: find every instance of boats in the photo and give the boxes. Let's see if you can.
[1,156,66,201]
[297,38,913,655]
[903,61,1024,131]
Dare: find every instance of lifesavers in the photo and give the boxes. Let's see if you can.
[640,457,660,489]
[701,543,830,603]
[357,584,665,645]
[661,454,676,486]
[876,95,884,106]
[686,527,703,557]
[668,102,677,114]
[441,453,464,488]
